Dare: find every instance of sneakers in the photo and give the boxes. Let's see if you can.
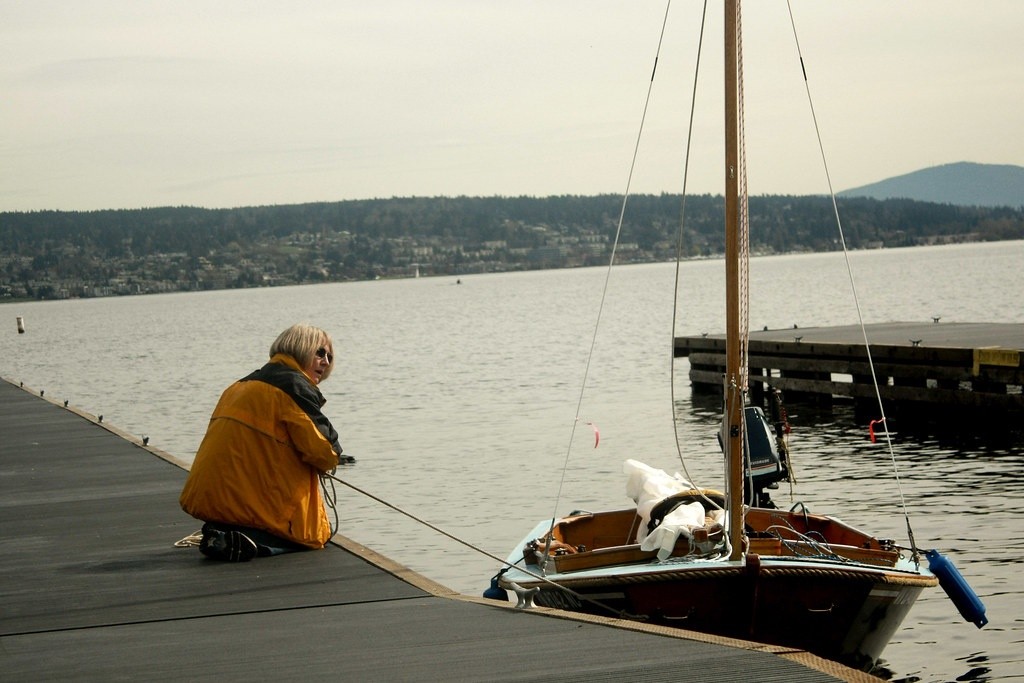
[199,529,258,563]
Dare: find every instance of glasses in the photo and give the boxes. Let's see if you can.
[316,347,333,364]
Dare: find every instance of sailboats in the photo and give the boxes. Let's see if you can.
[482,1,989,673]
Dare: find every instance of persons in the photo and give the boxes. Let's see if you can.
[177,320,347,565]
